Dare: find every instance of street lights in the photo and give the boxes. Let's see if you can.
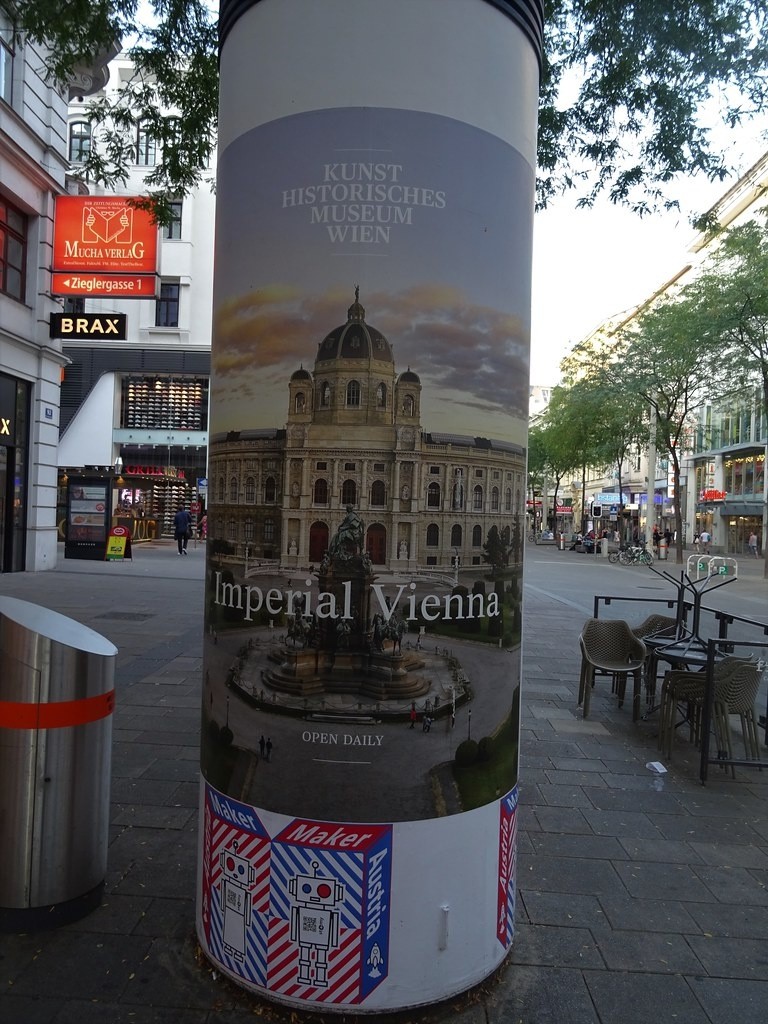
[227,696,230,727]
[467,710,472,740]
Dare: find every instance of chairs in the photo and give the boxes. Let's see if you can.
[579,614,768,779]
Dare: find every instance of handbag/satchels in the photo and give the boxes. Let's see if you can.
[707,535,711,541]
[185,526,193,538]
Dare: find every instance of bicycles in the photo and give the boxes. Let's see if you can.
[608,539,654,566]
[529,533,541,542]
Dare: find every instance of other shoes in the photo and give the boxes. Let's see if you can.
[147,484,197,533]
[178,553,182,555]
[182,548,188,555]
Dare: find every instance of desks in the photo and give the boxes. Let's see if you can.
[640,565,738,738]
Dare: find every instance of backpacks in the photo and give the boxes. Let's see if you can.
[695,538,700,544]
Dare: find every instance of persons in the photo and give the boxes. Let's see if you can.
[745,532,759,559]
[174,505,192,555]
[290,600,304,638]
[71,487,85,499]
[370,612,399,644]
[400,539,408,551]
[692,530,710,555]
[320,503,372,575]
[576,530,595,543]
[542,529,554,539]
[402,486,409,498]
[613,529,620,545]
[422,714,431,732]
[259,735,273,762]
[137,503,144,517]
[601,527,609,540]
[198,510,207,542]
[336,620,350,647]
[114,504,123,516]
[410,707,416,729]
[353,282,360,300]
[653,524,671,553]
[293,483,298,495]
